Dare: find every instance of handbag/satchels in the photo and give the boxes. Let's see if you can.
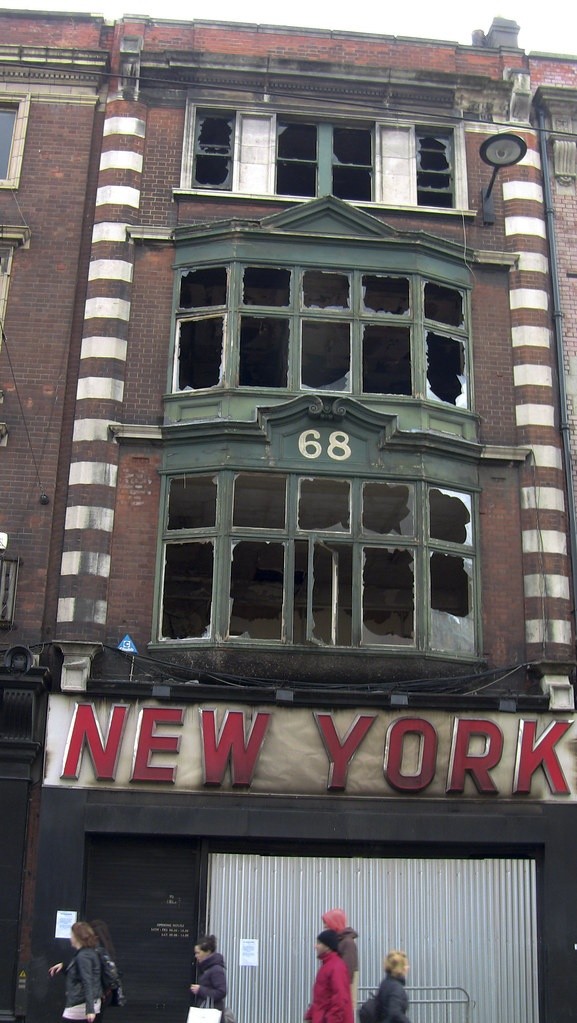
[186,995,222,1023]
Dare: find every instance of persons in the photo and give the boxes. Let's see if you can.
[322,907,361,1015]
[372,950,413,1023]
[189,934,228,1023]
[46,919,116,1023]
[304,930,356,1023]
[85,919,118,1023]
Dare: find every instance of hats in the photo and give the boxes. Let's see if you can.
[317,929,338,951]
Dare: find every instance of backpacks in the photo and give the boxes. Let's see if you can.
[358,980,400,1023]
